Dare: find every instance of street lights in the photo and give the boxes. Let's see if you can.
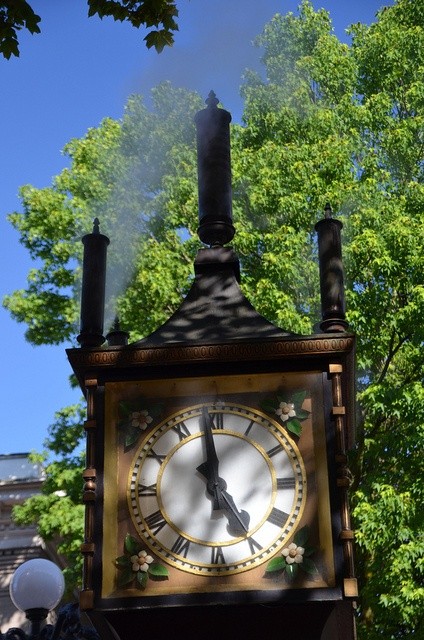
[1,559,93,640]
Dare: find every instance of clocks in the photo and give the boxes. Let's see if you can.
[64,91,363,639]
